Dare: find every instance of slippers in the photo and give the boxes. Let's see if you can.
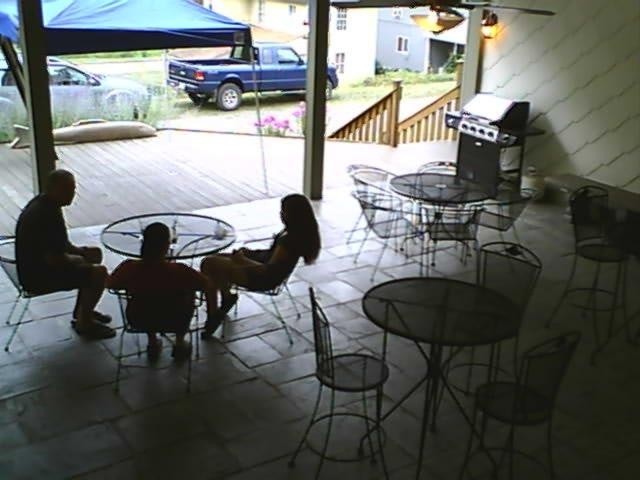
[172,341,192,357]
[201,311,225,338]
[147,339,163,356]
[220,293,238,315]
[77,322,115,338]
[71,312,112,327]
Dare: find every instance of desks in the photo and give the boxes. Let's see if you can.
[101,212,238,272]
[362,277,522,479]
[389,172,498,267]
[601,206,640,256]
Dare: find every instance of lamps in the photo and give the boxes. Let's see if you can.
[410,6,466,36]
[482,8,505,39]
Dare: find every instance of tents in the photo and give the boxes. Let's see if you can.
[1,1,269,198]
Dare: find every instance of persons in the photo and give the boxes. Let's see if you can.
[109,222,211,358]
[200,193,321,341]
[15,169,117,340]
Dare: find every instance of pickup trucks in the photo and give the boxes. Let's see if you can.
[166,42,339,111]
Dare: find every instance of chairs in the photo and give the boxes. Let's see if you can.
[414,162,476,248]
[460,329,583,480]
[221,233,301,346]
[418,201,487,283]
[0,256,83,352]
[460,187,536,266]
[350,190,423,282]
[108,287,203,395]
[429,242,542,434]
[288,286,392,479]
[347,163,425,252]
[544,184,631,352]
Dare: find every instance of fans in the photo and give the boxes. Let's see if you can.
[329,0,556,16]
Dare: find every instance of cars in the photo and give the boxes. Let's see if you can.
[0,53,152,143]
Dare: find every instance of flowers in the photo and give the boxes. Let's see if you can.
[254,101,306,139]
